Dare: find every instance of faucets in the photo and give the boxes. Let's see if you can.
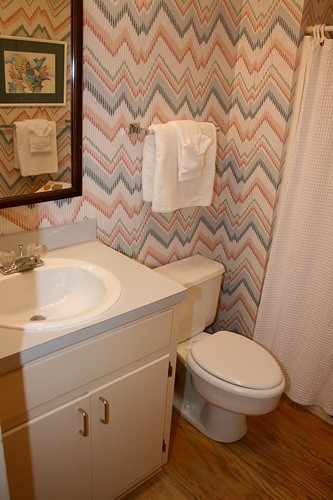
[1,256,44,274]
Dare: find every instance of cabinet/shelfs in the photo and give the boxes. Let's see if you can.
[0,302,181,500]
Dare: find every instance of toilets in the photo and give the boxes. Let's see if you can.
[151,254,287,445]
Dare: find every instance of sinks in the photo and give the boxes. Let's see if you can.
[0,257,120,330]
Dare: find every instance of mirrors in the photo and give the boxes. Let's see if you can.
[0,0,83,208]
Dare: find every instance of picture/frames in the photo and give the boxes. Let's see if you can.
[0,34,68,107]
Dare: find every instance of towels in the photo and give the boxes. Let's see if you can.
[25,118,55,155]
[168,120,211,182]
[142,122,216,214]
[13,121,58,177]
